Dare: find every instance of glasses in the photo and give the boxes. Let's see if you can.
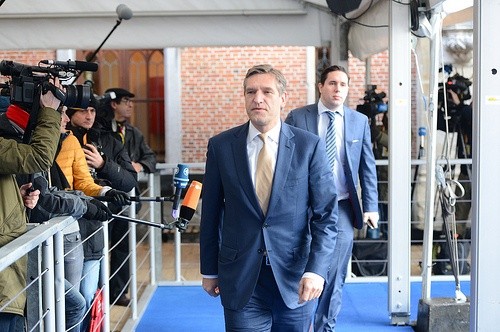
[115,99,132,103]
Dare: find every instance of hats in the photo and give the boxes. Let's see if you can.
[104,88,135,98]
[65,91,98,120]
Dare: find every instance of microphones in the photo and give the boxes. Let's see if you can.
[178,180,202,234]
[40,59,98,72]
[115,4,133,20]
[172,163,190,211]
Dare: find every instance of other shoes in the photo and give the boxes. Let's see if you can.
[109,291,131,307]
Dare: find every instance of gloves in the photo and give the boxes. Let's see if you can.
[93,208,110,222]
[95,201,112,214]
[108,189,132,206]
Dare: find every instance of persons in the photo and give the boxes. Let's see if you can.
[200,65,339,332]
[283,66,379,332]
[0,88,156,332]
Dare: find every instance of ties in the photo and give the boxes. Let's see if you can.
[323,111,339,174]
[254,133,275,218]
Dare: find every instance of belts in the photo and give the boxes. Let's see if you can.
[261,255,272,267]
